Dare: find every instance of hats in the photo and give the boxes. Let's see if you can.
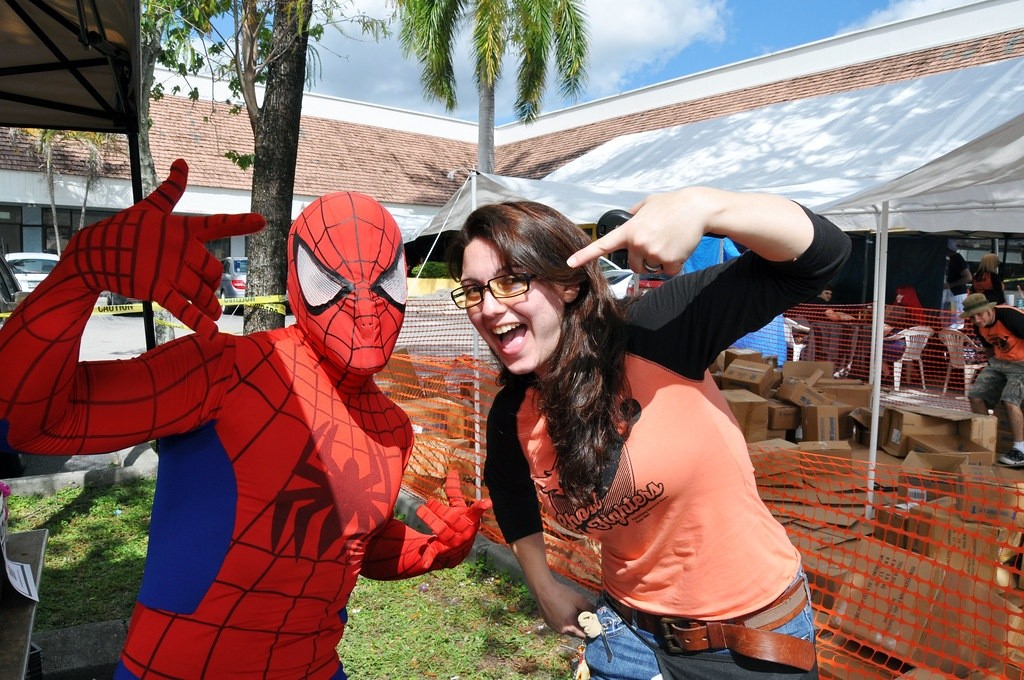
[959,293,996,318]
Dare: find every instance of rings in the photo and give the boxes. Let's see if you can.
[643,260,663,274]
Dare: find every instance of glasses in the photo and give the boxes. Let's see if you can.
[451,273,536,309]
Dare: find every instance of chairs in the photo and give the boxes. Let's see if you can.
[938,329,987,400]
[783,317,810,362]
[883,325,934,392]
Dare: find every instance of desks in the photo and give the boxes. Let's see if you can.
[0,529,49,680]
[808,322,871,362]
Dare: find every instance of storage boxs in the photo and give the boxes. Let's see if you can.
[372,344,1024,679]
[25,641,44,680]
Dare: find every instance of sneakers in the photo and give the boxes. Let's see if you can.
[999,448,1024,464]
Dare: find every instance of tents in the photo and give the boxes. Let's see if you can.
[399,53,1024,501]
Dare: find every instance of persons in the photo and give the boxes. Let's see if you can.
[447,185,851,680]
[944,239,1006,309]
[0,160,492,680]
[959,293,1024,465]
[805,285,926,385]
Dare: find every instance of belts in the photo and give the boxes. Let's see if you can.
[603,577,816,671]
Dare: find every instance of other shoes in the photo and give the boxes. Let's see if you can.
[833,366,851,377]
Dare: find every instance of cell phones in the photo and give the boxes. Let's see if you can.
[596,209,635,259]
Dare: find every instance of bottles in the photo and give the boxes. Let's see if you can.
[1017,295,1022,308]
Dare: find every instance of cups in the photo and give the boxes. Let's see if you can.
[1006,295,1014,306]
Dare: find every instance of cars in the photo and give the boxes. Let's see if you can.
[597,255,677,300]
[6,253,61,294]
[219,257,293,316]
[0,253,31,331]
